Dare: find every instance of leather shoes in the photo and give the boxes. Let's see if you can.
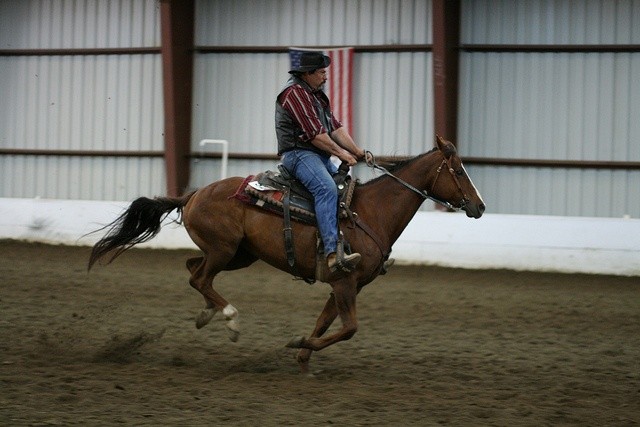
[379,257,395,275]
[328,251,361,272]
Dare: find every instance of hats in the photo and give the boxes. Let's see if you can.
[288,51,331,72]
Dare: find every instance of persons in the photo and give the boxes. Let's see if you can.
[274,51,372,275]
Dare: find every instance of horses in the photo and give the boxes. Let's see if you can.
[74,133,487,371]
